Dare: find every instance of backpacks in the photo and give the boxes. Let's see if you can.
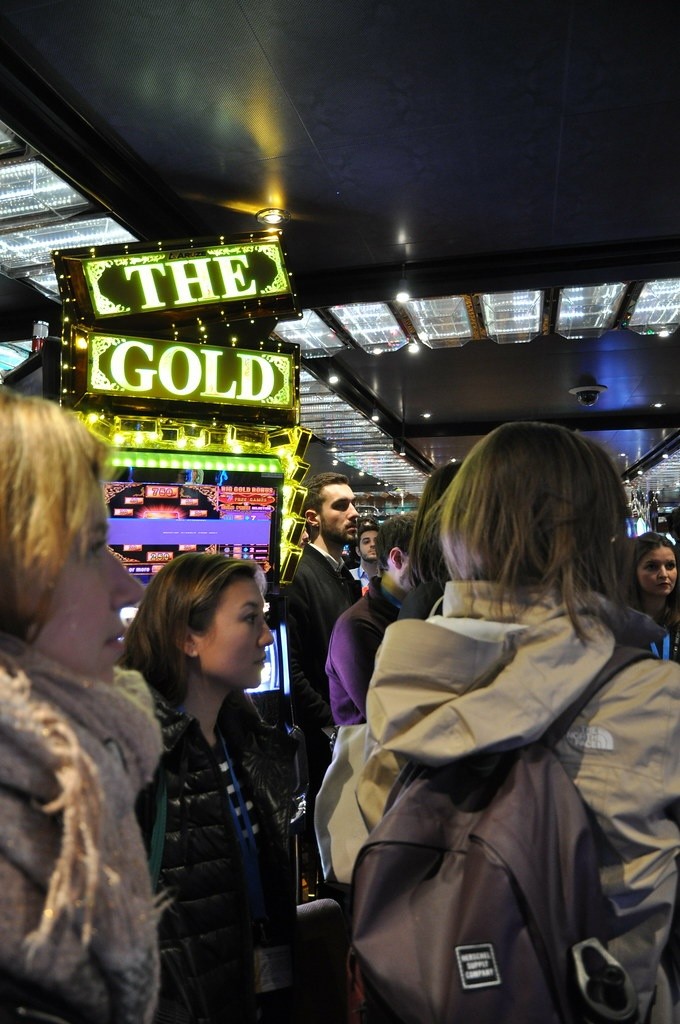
[345,645,660,1024]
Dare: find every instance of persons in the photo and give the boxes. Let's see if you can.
[0,396,680,1024]
